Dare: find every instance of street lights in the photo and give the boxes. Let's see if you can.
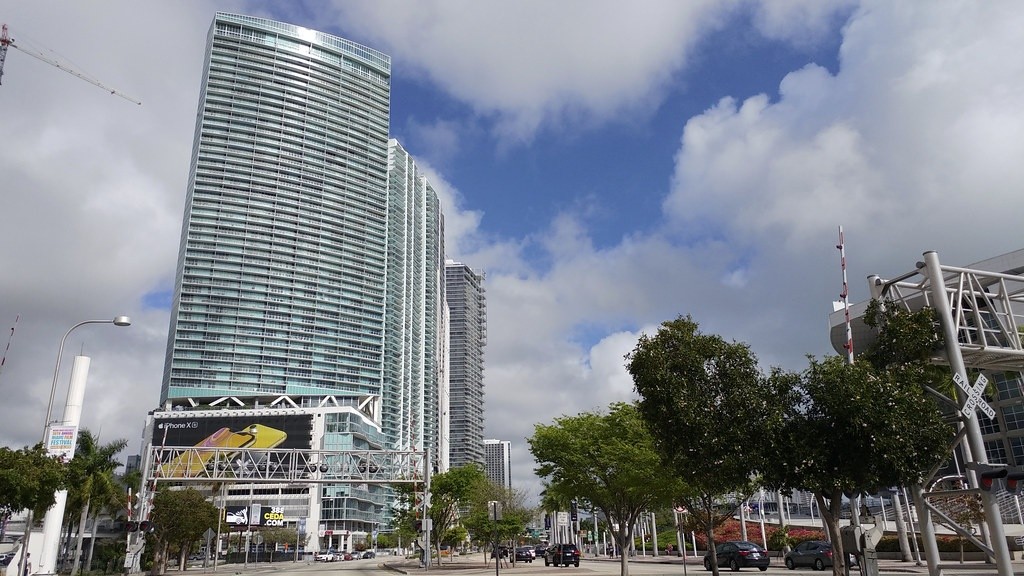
[17,315,132,576]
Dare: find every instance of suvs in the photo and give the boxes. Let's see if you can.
[545,543,580,568]
[534,544,551,558]
[498,545,509,558]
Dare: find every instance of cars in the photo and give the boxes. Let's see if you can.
[510,547,532,563]
[344,552,353,561]
[784,539,858,572]
[317,551,335,563]
[351,551,362,561]
[363,551,375,559]
[704,540,770,572]
[491,549,503,559]
[333,552,345,562]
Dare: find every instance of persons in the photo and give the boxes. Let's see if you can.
[17,553,32,576]
[665,543,673,555]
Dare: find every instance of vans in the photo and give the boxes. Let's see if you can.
[522,545,536,560]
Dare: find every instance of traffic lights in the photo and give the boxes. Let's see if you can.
[571,503,578,522]
[978,463,1024,493]
[546,532,551,540]
[416,519,424,534]
[125,520,150,533]
[544,514,550,530]
